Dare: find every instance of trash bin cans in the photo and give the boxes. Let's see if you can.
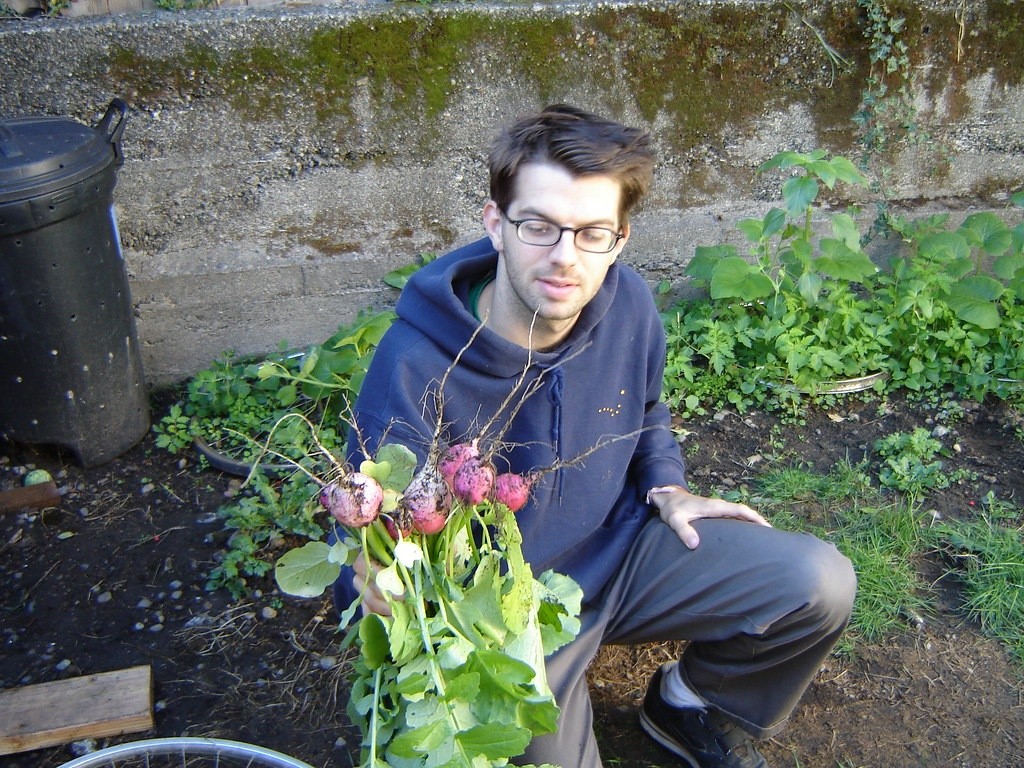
[0,96,158,469]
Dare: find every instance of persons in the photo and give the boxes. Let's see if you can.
[326,103,858,768]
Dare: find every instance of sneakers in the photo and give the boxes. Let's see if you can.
[637,659,768,768]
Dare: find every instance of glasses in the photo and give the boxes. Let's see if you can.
[497,204,627,253]
